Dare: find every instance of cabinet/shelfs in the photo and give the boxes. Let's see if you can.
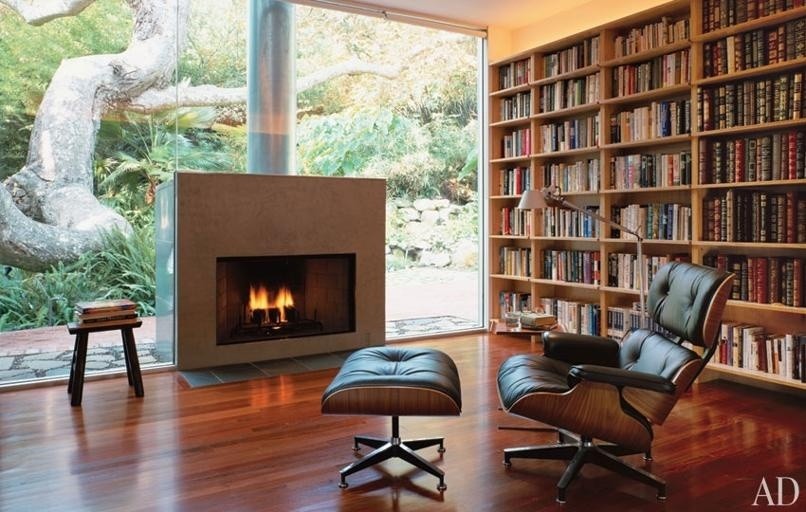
[488,0,805,400]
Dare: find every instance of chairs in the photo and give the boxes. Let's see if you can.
[498,260,738,504]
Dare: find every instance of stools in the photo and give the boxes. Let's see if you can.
[65,316,149,408]
[318,346,462,496]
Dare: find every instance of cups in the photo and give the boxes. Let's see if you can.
[504,311,523,333]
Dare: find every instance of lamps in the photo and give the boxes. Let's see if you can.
[516,185,645,332]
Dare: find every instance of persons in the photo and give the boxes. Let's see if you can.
[539,296,600,336]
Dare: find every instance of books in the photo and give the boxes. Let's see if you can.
[609,151,691,189]
[703,21,805,80]
[73,298,138,328]
[540,115,601,154]
[519,312,559,331]
[698,132,805,185]
[497,57,531,91]
[501,207,531,235]
[703,190,805,243]
[499,291,531,319]
[540,248,601,285]
[609,204,691,239]
[702,249,804,307]
[542,39,598,78]
[499,167,530,195]
[499,93,530,121]
[702,0,805,32]
[503,128,530,158]
[540,158,599,191]
[609,98,690,145]
[498,246,530,276]
[612,49,689,97]
[607,253,691,292]
[615,17,688,58]
[541,206,600,237]
[607,302,692,350]
[703,319,805,383]
[697,75,802,132]
[539,72,599,113]
